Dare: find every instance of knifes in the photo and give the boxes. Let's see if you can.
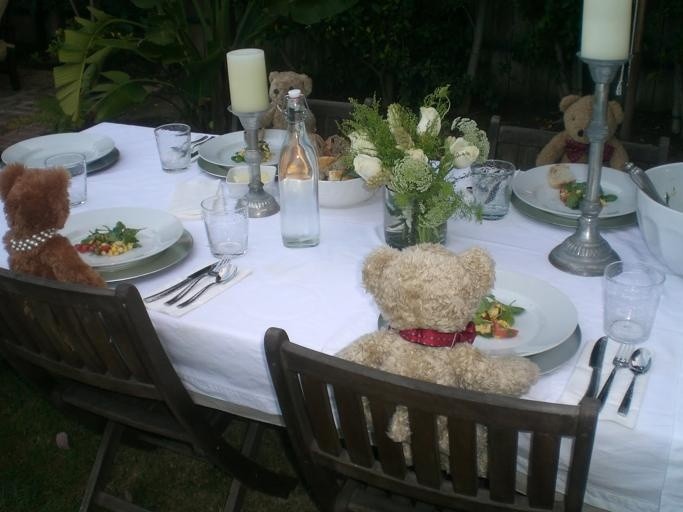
[578,334,608,408]
[144,256,231,305]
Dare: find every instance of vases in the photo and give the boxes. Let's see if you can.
[383,185,446,249]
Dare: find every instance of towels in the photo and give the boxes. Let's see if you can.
[168,178,225,221]
[142,271,251,316]
[558,339,656,428]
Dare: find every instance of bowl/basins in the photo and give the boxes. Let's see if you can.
[636,162,683,276]
[225,165,277,197]
[311,175,378,208]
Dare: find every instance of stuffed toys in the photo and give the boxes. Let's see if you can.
[534,94,630,171]
[323,242,540,478]
[0,163,108,366]
[260,70,316,135]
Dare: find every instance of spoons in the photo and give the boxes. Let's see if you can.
[618,346,653,419]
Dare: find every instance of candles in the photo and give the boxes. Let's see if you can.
[226,48,269,111]
[581,0,632,60]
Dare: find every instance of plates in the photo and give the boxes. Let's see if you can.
[376,268,583,379]
[4,133,121,176]
[512,161,637,232]
[61,205,195,284]
[198,130,291,181]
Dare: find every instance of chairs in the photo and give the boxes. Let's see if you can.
[489,116,670,173]
[231,97,374,141]
[266,327,601,512]
[1,267,300,512]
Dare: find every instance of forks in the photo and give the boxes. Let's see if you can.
[166,258,241,311]
[596,343,635,406]
[171,133,215,155]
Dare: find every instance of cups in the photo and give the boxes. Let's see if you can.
[600,259,666,343]
[200,197,249,257]
[471,157,515,221]
[44,151,88,206]
[154,124,191,171]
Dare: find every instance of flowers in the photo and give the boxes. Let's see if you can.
[326,84,490,242]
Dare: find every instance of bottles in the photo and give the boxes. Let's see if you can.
[277,89,321,247]
